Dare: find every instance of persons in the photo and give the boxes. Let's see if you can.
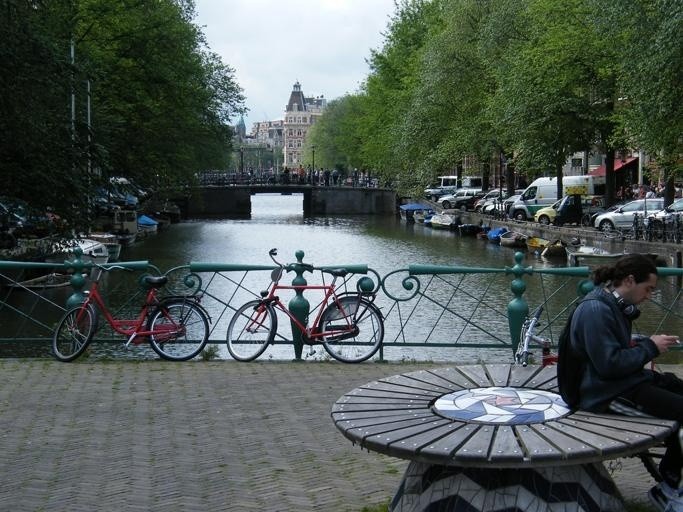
[565,251,683,512]
[246,164,358,187]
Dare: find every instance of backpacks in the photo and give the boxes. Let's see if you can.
[556,295,616,410]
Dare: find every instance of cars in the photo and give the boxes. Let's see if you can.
[0,175,151,244]
[423,172,682,232]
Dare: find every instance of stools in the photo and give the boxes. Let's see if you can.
[332,364,678,512]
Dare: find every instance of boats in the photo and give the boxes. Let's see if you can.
[5,271,89,292]
[395,202,627,270]
[48,202,180,264]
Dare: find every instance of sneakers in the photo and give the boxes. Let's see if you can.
[648,481,683,512]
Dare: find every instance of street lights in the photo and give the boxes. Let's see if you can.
[240,145,244,175]
[310,142,317,187]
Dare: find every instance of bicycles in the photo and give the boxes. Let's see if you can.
[630,210,682,244]
[222,245,389,364]
[511,302,665,483]
[47,260,215,363]
